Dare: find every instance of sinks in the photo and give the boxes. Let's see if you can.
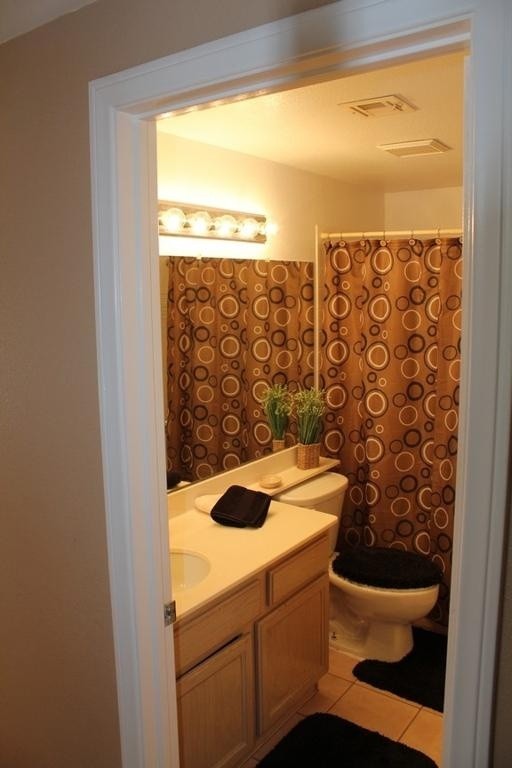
[169,549,214,596]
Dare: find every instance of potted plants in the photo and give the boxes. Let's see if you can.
[261,383,292,452]
[289,385,327,469]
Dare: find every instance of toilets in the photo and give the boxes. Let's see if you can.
[279,470,441,664]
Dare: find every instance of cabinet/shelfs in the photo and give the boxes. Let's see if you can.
[173,520,342,767]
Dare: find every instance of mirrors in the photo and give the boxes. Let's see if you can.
[163,252,320,495]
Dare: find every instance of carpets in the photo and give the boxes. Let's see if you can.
[256,711,438,766]
[351,622,449,712]
